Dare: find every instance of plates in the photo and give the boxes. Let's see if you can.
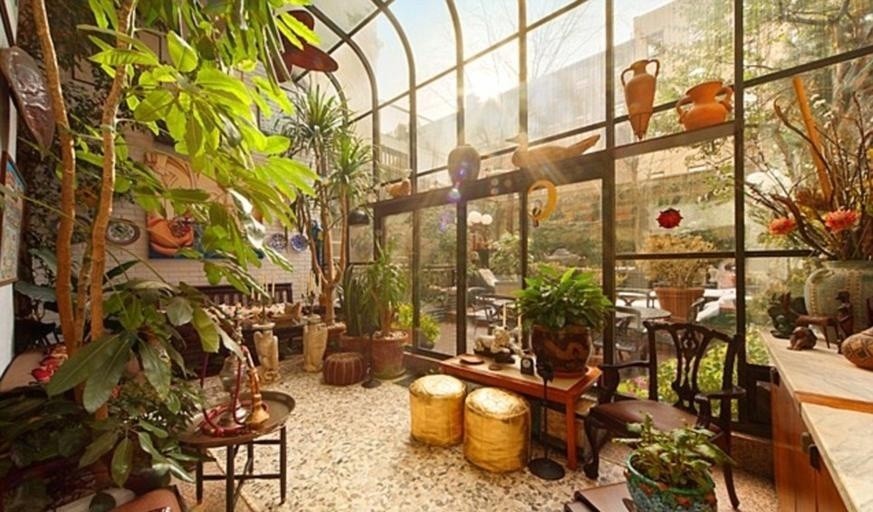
[52,214,91,244]
[290,234,308,252]
[271,233,288,252]
[106,218,140,246]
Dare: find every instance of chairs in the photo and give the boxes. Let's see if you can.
[581,317,745,510]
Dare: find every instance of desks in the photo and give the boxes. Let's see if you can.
[168,390,296,512]
[439,348,604,471]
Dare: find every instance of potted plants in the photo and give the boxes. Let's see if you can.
[722,75,873,344]
[337,262,369,355]
[629,230,720,326]
[610,410,739,512]
[513,259,619,380]
[268,68,381,366]
[364,232,411,379]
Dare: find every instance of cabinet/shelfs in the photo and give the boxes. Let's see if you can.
[751,329,850,512]
